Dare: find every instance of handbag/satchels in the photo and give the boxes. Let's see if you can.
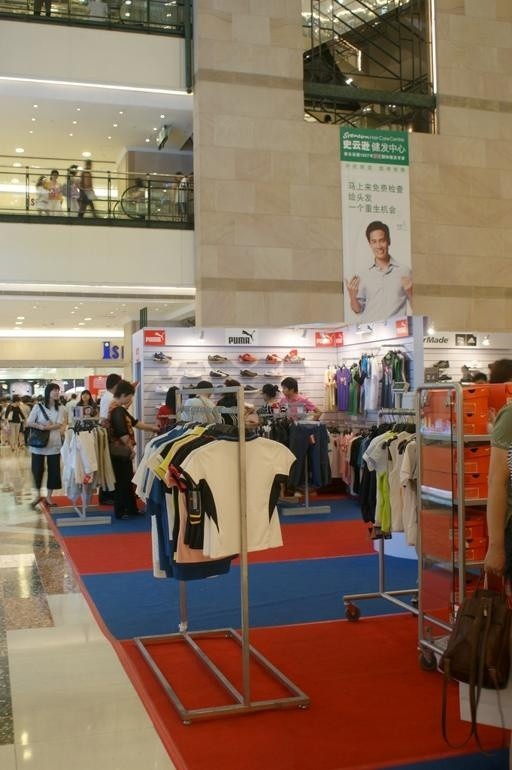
[23,423,52,449]
[439,568,512,691]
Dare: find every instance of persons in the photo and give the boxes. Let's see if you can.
[345,220,414,324]
[0,372,322,519]
[35,159,194,224]
[483,400,511,609]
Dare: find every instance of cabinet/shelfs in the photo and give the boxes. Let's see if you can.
[414,380,512,675]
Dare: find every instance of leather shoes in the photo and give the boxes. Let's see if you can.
[28,496,43,507]
[44,497,57,508]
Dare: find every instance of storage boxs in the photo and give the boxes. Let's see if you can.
[419,382,512,626]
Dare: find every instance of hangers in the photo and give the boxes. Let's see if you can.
[259,408,417,438]
[69,418,103,436]
[327,344,403,371]
[175,405,254,438]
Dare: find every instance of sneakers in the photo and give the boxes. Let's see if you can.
[265,353,282,363]
[0,437,24,451]
[98,492,147,520]
[284,350,306,364]
[207,354,228,361]
[264,369,281,376]
[240,369,258,378]
[238,352,257,362]
[433,359,449,369]
[463,360,482,369]
[209,368,229,378]
[244,384,259,393]
[154,384,169,393]
[152,352,173,361]
